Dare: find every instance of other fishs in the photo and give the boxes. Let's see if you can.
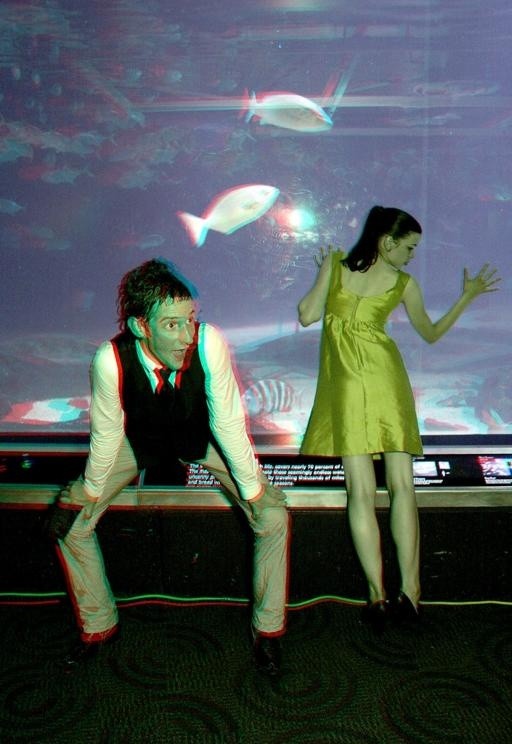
[243,90,330,137]
[1,0,184,218]
[173,182,280,250]
[237,376,306,419]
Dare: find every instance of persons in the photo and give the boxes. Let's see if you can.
[41,257,295,679]
[296,203,504,634]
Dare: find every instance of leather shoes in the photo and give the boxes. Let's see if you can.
[63,632,121,675]
[254,637,281,678]
[389,591,440,633]
[362,600,388,636]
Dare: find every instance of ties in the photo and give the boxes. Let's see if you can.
[155,365,175,394]
